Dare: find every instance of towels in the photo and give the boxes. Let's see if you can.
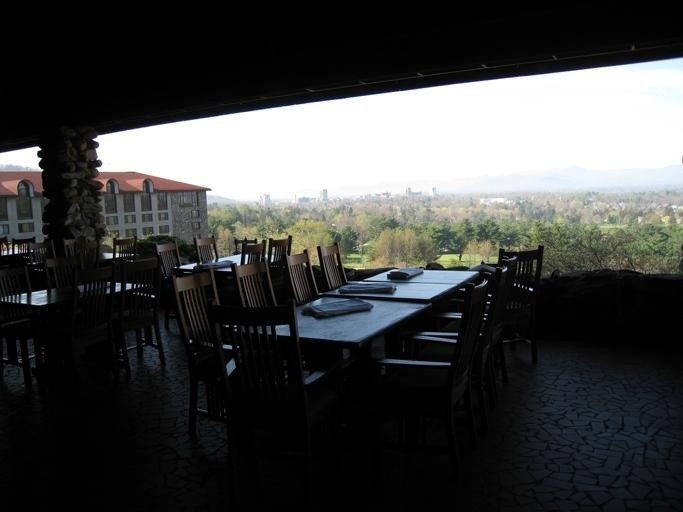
[301,297,374,320]
[387,268,423,280]
[339,283,397,294]
[193,260,233,271]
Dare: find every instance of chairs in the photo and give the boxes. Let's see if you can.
[207,304,326,454]
[45,260,119,413]
[498,244,543,365]
[243,241,265,271]
[170,269,241,439]
[268,237,294,268]
[286,250,319,306]
[417,267,498,413]
[158,241,181,269]
[194,235,220,261]
[318,245,348,292]
[1,257,30,379]
[111,238,137,267]
[33,240,54,262]
[36,254,86,287]
[234,238,251,256]
[418,257,516,402]
[73,245,100,275]
[13,239,39,280]
[231,257,278,353]
[109,259,166,381]
[367,280,490,451]
